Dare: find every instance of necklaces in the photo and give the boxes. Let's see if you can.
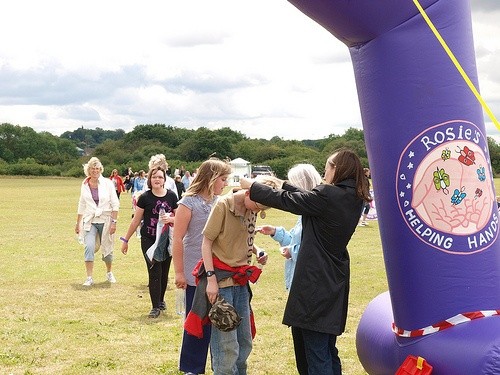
[255,202,266,219]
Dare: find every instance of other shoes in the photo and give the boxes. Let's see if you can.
[362,221,368,226]
[83,277,94,286]
[105,272,116,283]
[159,301,167,310]
[148,308,160,318]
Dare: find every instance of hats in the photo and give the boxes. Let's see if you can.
[208,295,243,332]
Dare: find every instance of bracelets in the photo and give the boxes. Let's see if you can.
[112,219,117,224]
[75,222,79,225]
[120,237,128,242]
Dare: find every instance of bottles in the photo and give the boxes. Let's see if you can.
[159,206,166,225]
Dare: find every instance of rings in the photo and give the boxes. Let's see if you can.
[183,285,185,286]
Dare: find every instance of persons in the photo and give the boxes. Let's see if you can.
[121,167,178,318]
[201,184,276,375]
[123,169,147,192]
[496,196,500,210]
[172,159,231,374]
[109,169,126,199]
[361,168,373,227]
[142,154,178,287]
[167,165,199,200]
[240,151,372,375]
[259,163,322,374]
[75,157,119,286]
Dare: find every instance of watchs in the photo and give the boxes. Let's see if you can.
[205,271,215,277]
